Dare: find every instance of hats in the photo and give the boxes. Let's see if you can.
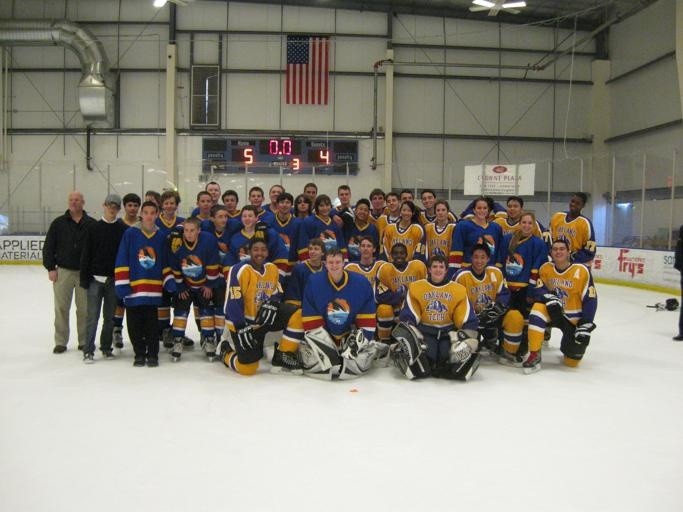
[105,195,121,207]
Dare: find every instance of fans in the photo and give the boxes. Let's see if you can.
[468,0,525,18]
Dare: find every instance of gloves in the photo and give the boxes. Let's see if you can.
[80,272,90,289]
[259,301,281,329]
[542,292,564,323]
[236,322,259,352]
[477,303,500,330]
[574,321,596,344]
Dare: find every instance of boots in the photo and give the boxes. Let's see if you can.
[270,342,303,369]
[159,326,234,366]
[112,325,124,348]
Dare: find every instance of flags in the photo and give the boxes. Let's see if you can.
[286,34,329,107]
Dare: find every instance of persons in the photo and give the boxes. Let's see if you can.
[43,182,551,382]
[542,192,597,273]
[522,240,598,367]
[673,226,683,341]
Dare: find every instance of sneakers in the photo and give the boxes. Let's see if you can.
[499,351,523,362]
[134,352,158,366]
[53,344,114,363]
[523,350,541,367]
